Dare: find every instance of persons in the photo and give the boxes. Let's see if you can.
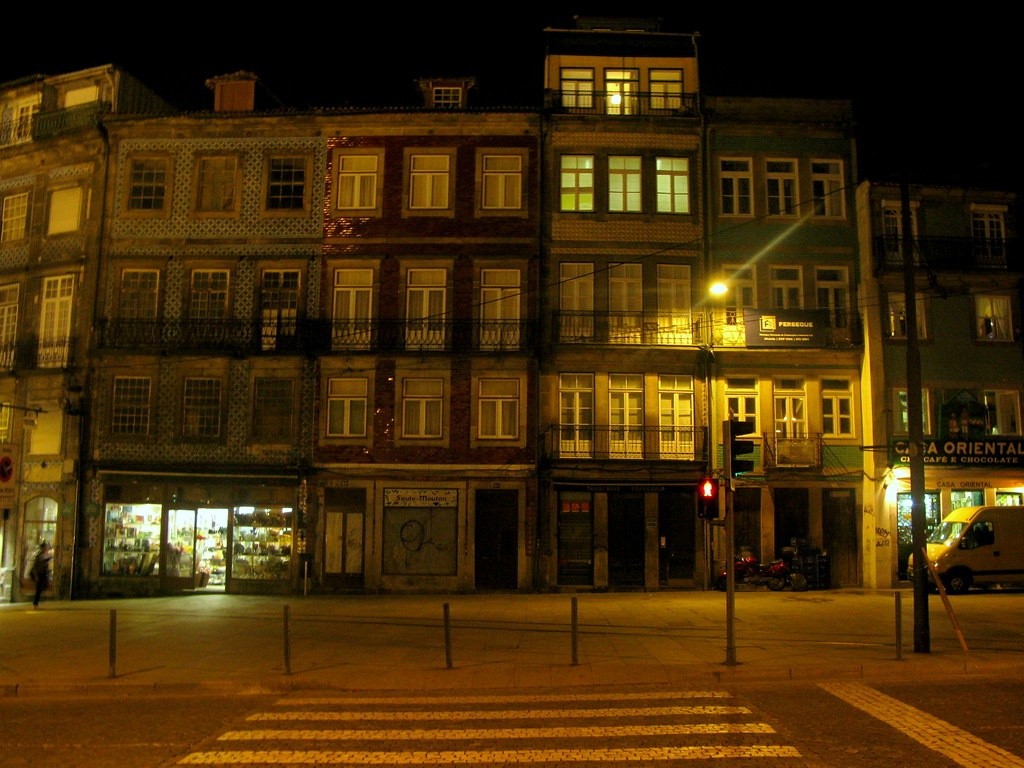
[29,542,52,610]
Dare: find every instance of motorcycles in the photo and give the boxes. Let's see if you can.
[715,552,790,592]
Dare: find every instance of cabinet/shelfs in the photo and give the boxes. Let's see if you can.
[204,547,226,585]
[233,524,292,577]
[105,522,161,555]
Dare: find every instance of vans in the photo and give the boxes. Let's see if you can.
[907,505,1024,596]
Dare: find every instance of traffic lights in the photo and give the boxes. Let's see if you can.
[723,418,755,491]
[697,478,719,518]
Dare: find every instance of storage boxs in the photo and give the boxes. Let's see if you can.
[105,506,143,538]
